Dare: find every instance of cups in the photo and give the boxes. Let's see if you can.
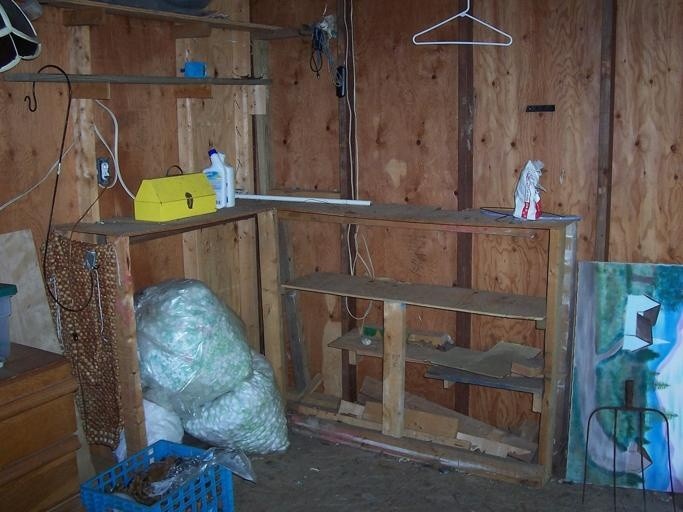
[184,61,205,78]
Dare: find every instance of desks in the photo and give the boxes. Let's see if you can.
[57,199,276,486]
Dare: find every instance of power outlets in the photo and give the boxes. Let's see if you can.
[97,159,112,188]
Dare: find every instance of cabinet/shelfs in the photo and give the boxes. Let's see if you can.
[0,344,80,512]
[278,202,581,490]
[0,1,282,85]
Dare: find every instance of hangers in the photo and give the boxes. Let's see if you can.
[412,1,513,47]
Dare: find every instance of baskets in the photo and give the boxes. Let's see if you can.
[80,440,235,512]
